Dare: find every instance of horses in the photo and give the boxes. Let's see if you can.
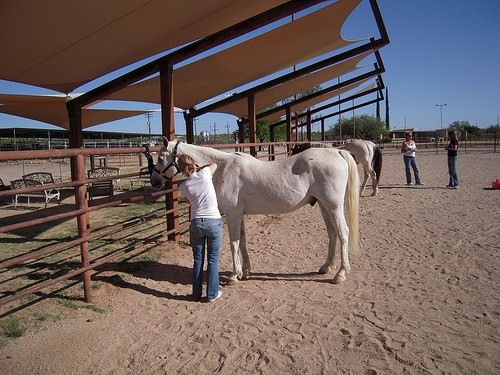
[149,135,362,286]
[291,140,385,197]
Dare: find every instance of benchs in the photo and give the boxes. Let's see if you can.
[9,178,61,210]
[130,164,159,190]
[86,176,116,204]
[22,172,65,194]
[87,167,122,191]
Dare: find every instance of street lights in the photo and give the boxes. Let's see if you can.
[435,103,447,129]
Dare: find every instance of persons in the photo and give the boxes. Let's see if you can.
[400,132,425,186]
[445,130,461,188]
[177,154,223,302]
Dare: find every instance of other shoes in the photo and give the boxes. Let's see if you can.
[408,182,413,185]
[446,184,454,187]
[454,184,458,189]
[417,182,424,184]
[208,291,222,302]
